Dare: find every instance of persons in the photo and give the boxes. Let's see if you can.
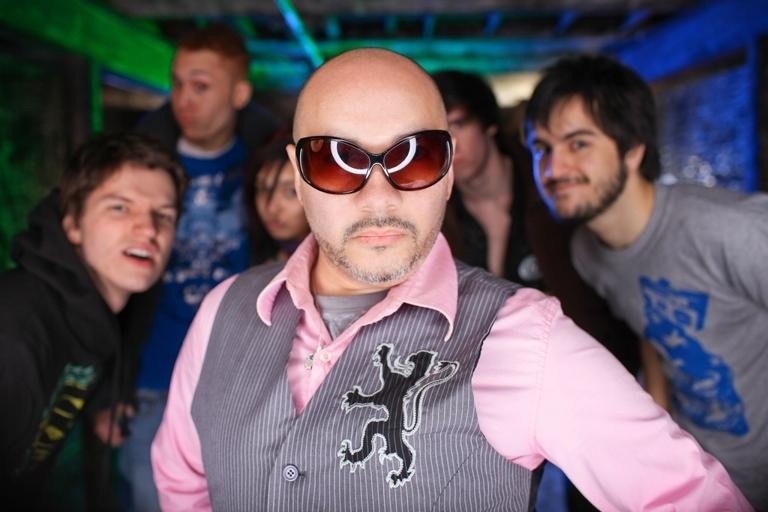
[523,56,768,512]
[83,20,281,512]
[239,118,314,266]
[425,68,642,377]
[1,130,189,512]
[149,48,753,512]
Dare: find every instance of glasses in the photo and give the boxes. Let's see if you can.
[295,129,455,195]
